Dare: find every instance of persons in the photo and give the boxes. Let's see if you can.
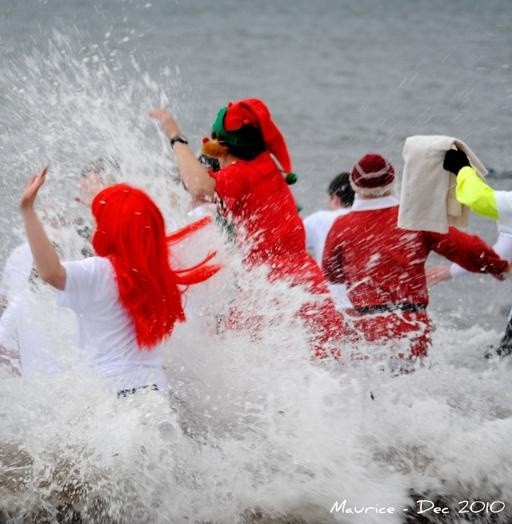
[424,230,511,288]
[1,242,86,380]
[18,163,224,440]
[321,151,511,359]
[147,96,340,357]
[442,142,512,357]
[1,199,86,320]
[58,154,143,260]
[301,172,355,311]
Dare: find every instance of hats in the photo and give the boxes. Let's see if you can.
[348,151,397,197]
[210,99,298,186]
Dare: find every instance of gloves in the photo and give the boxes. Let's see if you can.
[441,148,470,176]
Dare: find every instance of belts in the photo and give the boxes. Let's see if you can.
[356,303,426,314]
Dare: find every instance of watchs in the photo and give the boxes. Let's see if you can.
[168,132,188,149]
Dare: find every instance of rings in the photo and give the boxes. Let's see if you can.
[431,275,438,280]
